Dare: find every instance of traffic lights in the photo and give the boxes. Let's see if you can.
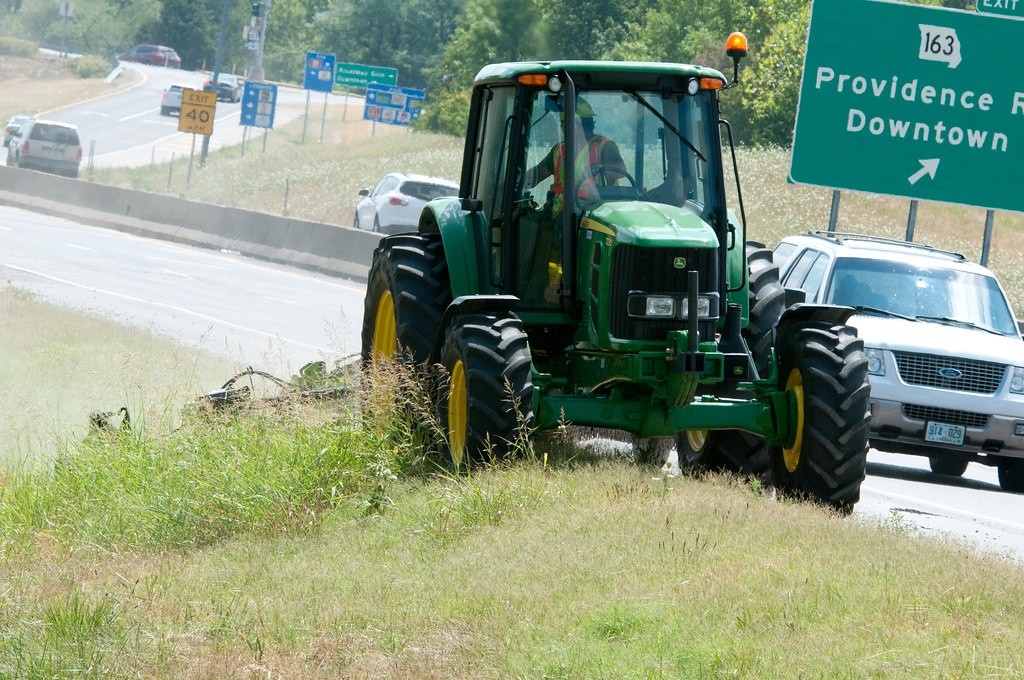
[251,3,260,16]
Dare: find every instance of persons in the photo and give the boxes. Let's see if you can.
[522,96,626,226]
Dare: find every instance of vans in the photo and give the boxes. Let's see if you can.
[7,119,81,177]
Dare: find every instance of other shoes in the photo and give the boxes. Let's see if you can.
[544,283,560,304]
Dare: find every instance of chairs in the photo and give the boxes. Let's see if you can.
[917,290,955,319]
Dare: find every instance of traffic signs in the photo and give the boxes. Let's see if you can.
[335,61,399,91]
[785,1,1024,210]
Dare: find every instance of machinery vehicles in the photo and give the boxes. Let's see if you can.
[359,30,871,515]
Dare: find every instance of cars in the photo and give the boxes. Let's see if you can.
[203,74,241,102]
[161,84,197,115]
[115,44,181,68]
[4,115,37,146]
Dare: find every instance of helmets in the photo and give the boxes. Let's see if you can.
[560,96,597,122]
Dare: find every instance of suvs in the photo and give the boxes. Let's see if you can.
[772,231,1024,495]
[353,170,461,236]
